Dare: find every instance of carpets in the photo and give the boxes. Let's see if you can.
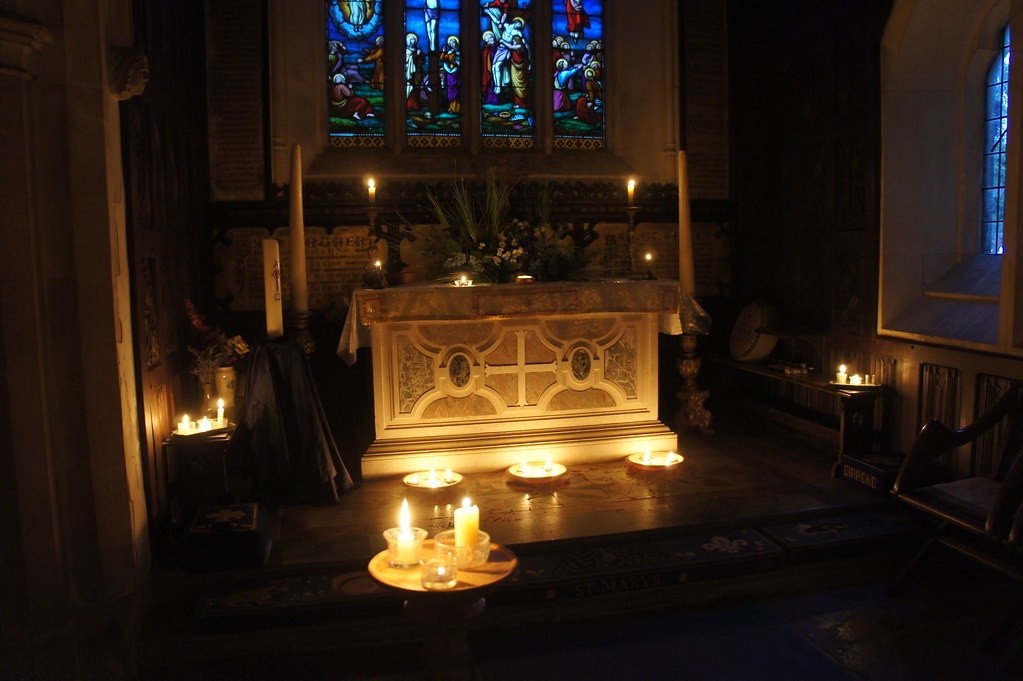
[130,503,1023,681]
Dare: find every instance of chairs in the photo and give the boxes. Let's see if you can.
[887,379,1023,654]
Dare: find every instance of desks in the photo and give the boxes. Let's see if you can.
[162,395,242,531]
[710,352,885,476]
[359,278,679,479]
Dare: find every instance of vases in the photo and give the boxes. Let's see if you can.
[199,384,216,417]
[215,367,237,407]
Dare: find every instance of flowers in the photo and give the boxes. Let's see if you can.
[379,164,587,284]
[184,298,249,367]
[187,345,222,384]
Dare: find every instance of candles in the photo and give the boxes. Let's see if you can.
[454,279,473,286]
[366,177,376,206]
[176,413,196,435]
[837,373,847,384]
[397,530,414,562]
[849,376,861,384]
[262,238,283,339]
[627,184,635,206]
[454,504,479,559]
[197,414,215,432]
[216,398,225,427]
